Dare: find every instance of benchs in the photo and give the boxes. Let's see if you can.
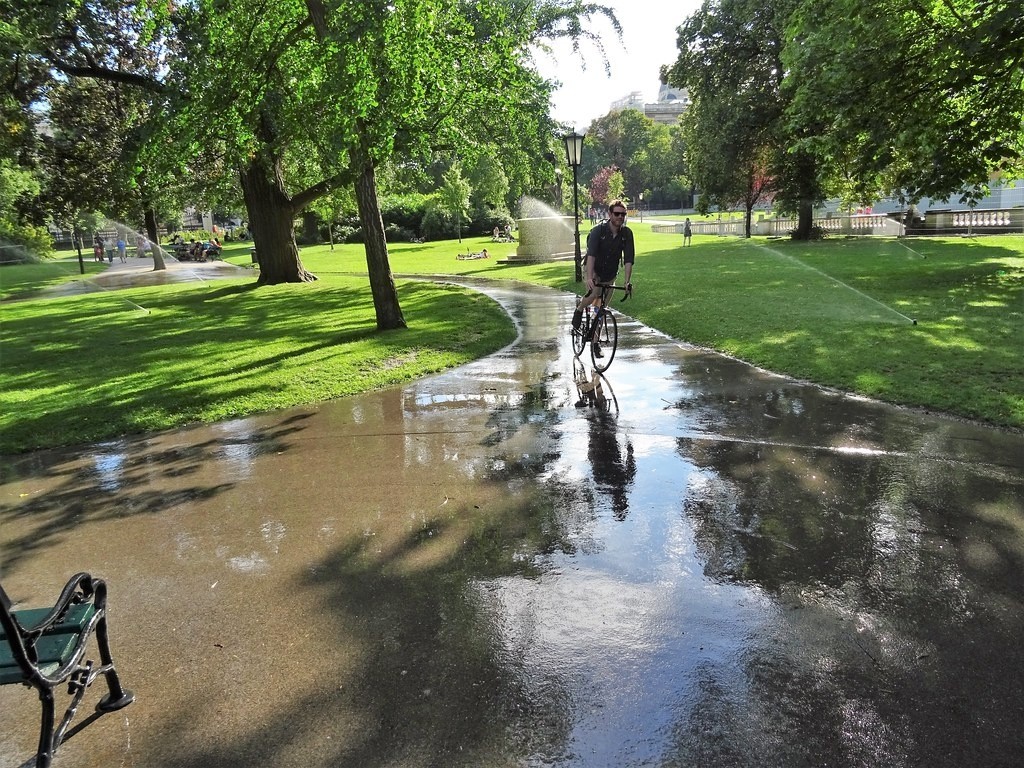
[0,572,135,768]
[204,247,222,262]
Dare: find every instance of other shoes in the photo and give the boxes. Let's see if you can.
[572,308,583,330]
[591,343,604,358]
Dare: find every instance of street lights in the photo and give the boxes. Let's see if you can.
[562,127,586,282]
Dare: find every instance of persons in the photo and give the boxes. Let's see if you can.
[682,218,692,247]
[492,225,515,243]
[224,232,235,242]
[93,232,104,261]
[116,237,127,263]
[589,207,606,225]
[171,234,222,264]
[856,203,864,215]
[103,237,115,263]
[136,237,151,258]
[237,231,248,241]
[569,200,634,358]
[458,248,488,260]
[212,225,218,233]
[866,205,873,215]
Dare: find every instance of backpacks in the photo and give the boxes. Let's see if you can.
[684,221,690,235]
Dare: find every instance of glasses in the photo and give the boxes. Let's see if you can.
[611,212,627,217]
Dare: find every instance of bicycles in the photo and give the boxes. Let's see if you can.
[571,279,633,372]
